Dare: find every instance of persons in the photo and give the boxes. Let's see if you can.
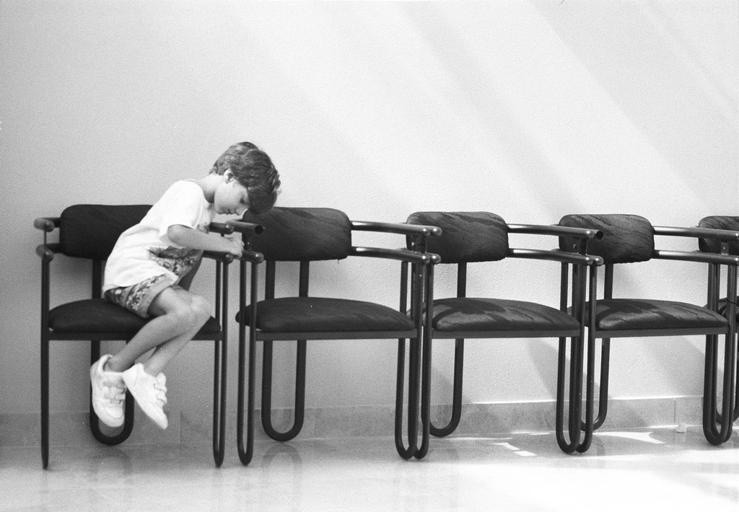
[90,142,281,430]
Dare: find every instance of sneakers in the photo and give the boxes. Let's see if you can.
[90,353,126,428]
[120,363,169,430]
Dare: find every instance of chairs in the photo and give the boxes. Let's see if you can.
[226,204,431,468]
[35,204,236,470]
[406,211,737,457]
